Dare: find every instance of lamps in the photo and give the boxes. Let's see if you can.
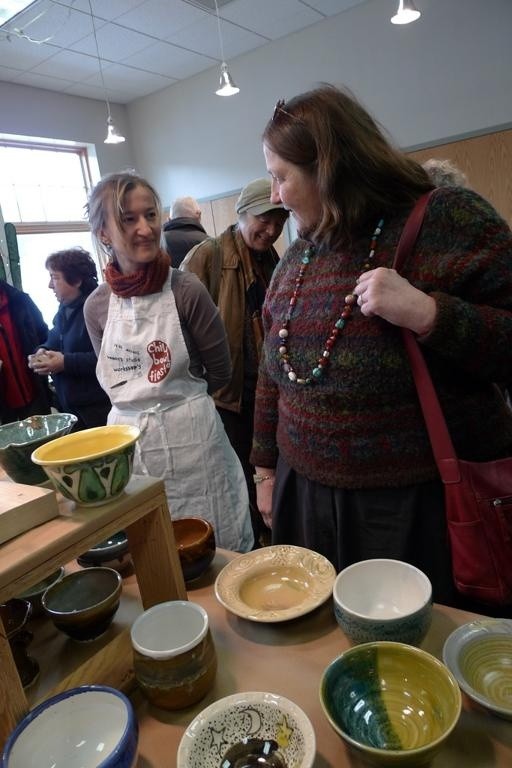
[89,1,125,145]
[390,0,421,27]
[215,0,240,96]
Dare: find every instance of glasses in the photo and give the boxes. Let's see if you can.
[272,99,306,126]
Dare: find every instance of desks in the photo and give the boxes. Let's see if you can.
[24,547,512,768]
[1,468,185,745]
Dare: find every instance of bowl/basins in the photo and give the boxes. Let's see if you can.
[216,544,337,622]
[30,424,140,509]
[170,515,215,585]
[77,530,132,571]
[28,353,51,365]
[332,556,433,648]
[1,413,76,486]
[42,567,122,644]
[318,640,462,766]
[11,566,64,611]
[441,618,511,720]
[175,691,317,768]
[2,684,140,767]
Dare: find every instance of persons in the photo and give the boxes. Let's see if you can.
[161,195,210,267]
[28,247,112,433]
[177,177,291,546]
[249,81,512,619]
[421,157,472,190]
[82,167,256,554]
[0,280,61,425]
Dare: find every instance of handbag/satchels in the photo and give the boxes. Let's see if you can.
[441,455,512,603]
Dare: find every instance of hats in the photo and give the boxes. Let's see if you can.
[235,179,284,217]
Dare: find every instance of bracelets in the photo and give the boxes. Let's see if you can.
[252,471,273,483]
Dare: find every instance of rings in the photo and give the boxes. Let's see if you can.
[49,371,52,375]
[358,294,364,304]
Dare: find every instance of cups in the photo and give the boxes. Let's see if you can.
[130,600,218,710]
[0,597,42,689]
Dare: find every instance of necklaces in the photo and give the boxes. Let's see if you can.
[279,216,386,384]
